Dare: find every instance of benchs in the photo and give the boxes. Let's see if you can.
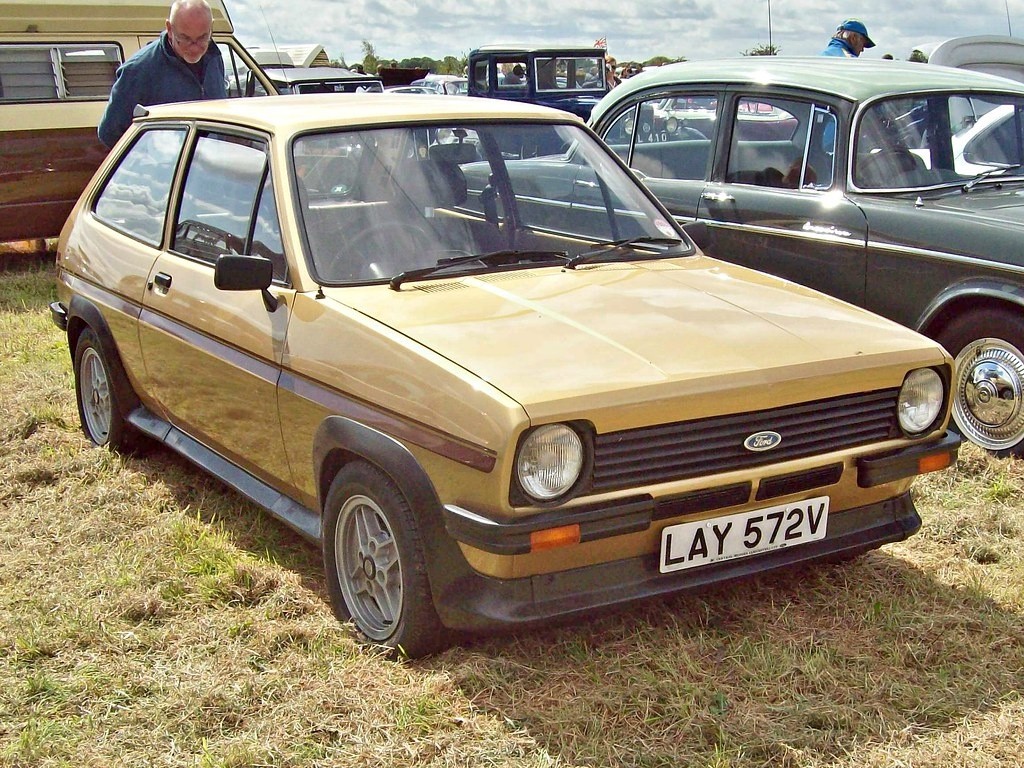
[608,140,802,185]
[197,201,389,269]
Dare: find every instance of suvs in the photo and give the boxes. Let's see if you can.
[467,45,713,157]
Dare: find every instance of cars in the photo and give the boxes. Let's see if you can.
[224,43,467,96]
[868,104,1024,177]
[655,95,799,141]
[456,54,1024,460]
[48,95,965,661]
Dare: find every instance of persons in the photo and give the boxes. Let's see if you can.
[816,17,876,153]
[390,63,397,69]
[371,64,384,87]
[906,50,929,149]
[350,65,367,76]
[503,64,524,85]
[97,0,227,149]
[724,158,817,189]
[583,56,622,91]
[617,62,644,79]
[463,66,469,89]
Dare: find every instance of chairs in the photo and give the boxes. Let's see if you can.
[380,160,504,270]
[253,175,364,280]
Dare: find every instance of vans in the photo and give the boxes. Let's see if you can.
[0,1,519,243]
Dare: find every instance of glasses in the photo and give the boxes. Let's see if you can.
[611,65,617,69]
[172,30,210,46]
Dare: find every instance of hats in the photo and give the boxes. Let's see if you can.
[837,18,876,48]
[349,64,363,71]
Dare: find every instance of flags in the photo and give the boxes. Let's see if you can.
[593,37,607,49]
